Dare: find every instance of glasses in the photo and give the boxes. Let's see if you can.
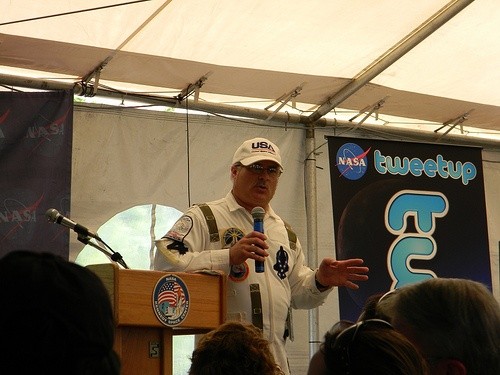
[335,318,395,375]
[237,164,283,177]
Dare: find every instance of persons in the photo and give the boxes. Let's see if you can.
[188,321,284,375]
[370,280,500,375]
[0,251,120,375]
[307,320,427,375]
[156,137,367,375]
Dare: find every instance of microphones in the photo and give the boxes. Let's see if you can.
[251,206,266,273]
[43,208,101,241]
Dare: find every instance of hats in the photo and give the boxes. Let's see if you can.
[232,138,284,170]
[1,249,122,374]
